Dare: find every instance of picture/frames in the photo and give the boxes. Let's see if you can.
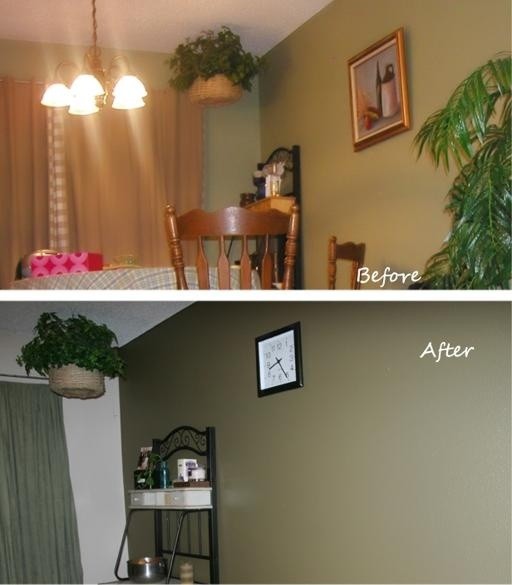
[346,27,410,152]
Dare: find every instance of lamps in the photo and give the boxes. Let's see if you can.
[41,0,147,115]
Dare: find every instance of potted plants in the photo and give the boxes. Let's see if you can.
[16,312,129,400]
[164,25,265,107]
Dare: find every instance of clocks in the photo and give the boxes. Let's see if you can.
[255,321,304,398]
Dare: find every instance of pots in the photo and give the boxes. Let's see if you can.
[127,558,167,584]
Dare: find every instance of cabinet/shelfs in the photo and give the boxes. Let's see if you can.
[101,425,220,585]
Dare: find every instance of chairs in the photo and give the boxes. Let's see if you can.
[327,235,365,289]
[165,200,300,289]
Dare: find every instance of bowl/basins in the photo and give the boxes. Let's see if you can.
[113,254,141,267]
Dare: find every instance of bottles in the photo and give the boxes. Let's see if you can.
[252,163,265,200]
[158,460,170,489]
[376,61,382,115]
[381,65,400,119]
[264,160,282,198]
[180,559,194,584]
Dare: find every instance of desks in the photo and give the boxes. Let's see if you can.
[10,266,260,290]
